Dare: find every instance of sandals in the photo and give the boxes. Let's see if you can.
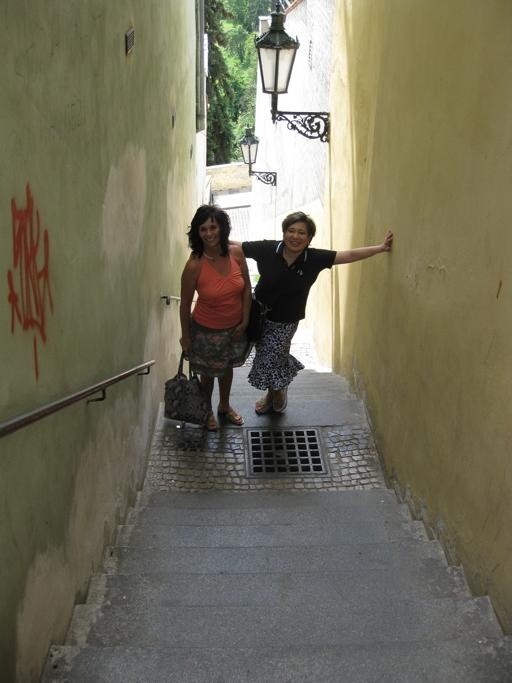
[254,385,288,415]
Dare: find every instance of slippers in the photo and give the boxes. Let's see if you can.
[219,407,245,426]
[206,413,217,431]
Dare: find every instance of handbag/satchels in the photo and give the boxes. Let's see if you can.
[163,374,213,427]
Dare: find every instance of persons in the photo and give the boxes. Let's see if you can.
[227,212,393,414]
[180,204,252,431]
